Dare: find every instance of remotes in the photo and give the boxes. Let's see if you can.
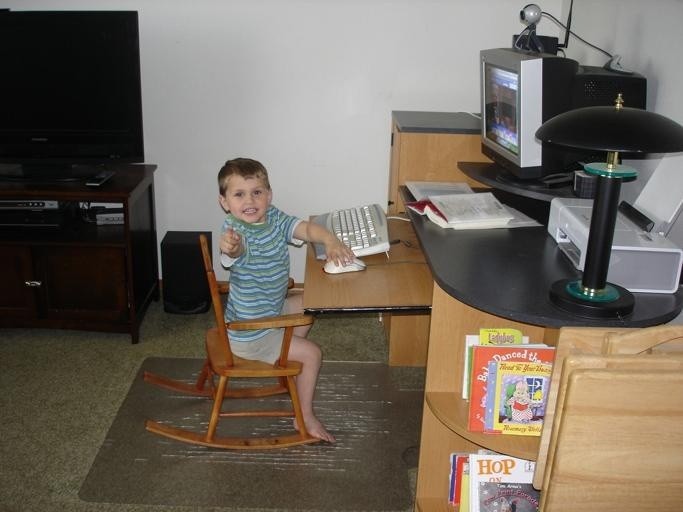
[86,170,115,186]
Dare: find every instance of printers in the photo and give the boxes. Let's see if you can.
[547,154,683,294]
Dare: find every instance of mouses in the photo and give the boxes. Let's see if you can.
[324,259,365,274]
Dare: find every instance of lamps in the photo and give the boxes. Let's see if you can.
[535,90,682,322]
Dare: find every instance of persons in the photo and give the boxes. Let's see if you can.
[215,156,356,444]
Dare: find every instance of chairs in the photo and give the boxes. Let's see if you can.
[142,234,324,449]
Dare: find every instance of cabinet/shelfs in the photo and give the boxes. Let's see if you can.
[0,163,160,345]
[385,110,495,367]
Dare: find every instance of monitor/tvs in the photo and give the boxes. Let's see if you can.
[0,10,144,181]
[479,50,646,191]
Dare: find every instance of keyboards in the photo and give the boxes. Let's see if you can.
[327,203,390,259]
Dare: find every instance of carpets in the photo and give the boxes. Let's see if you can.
[76,356,426,512]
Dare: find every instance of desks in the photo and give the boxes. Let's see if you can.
[302,183,683,512]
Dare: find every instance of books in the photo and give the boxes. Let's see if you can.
[450,323,557,512]
[402,176,543,230]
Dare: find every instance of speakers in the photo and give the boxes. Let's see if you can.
[160,229,212,315]
